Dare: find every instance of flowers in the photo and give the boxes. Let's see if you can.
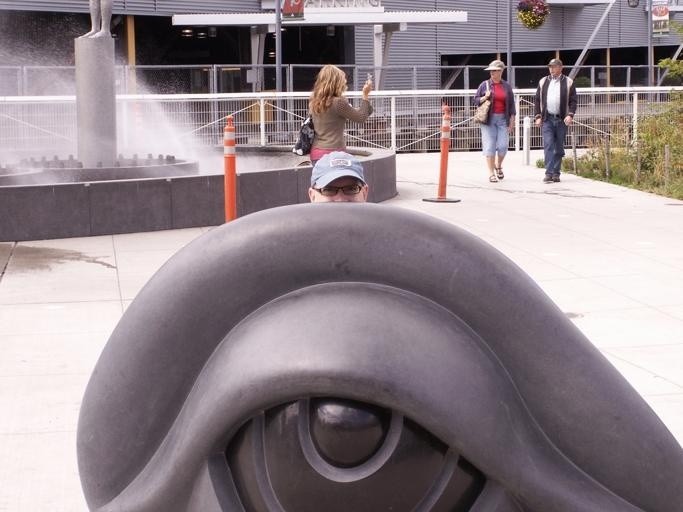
[515,0,550,32]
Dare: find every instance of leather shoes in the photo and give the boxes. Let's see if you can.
[551,175,559,181]
[543,175,551,180]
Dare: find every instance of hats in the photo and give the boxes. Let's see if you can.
[547,58,562,65]
[483,59,504,70]
[310,151,366,190]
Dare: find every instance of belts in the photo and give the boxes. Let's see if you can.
[548,114,558,117]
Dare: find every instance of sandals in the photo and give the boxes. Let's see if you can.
[494,164,503,178]
[489,174,496,182]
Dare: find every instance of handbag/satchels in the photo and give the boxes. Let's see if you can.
[473,80,490,124]
[292,114,314,156]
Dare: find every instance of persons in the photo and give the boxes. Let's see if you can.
[474,60,516,182]
[307,151,370,204]
[308,65,373,165]
[534,58,578,182]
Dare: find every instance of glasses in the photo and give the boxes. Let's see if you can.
[312,184,363,196]
